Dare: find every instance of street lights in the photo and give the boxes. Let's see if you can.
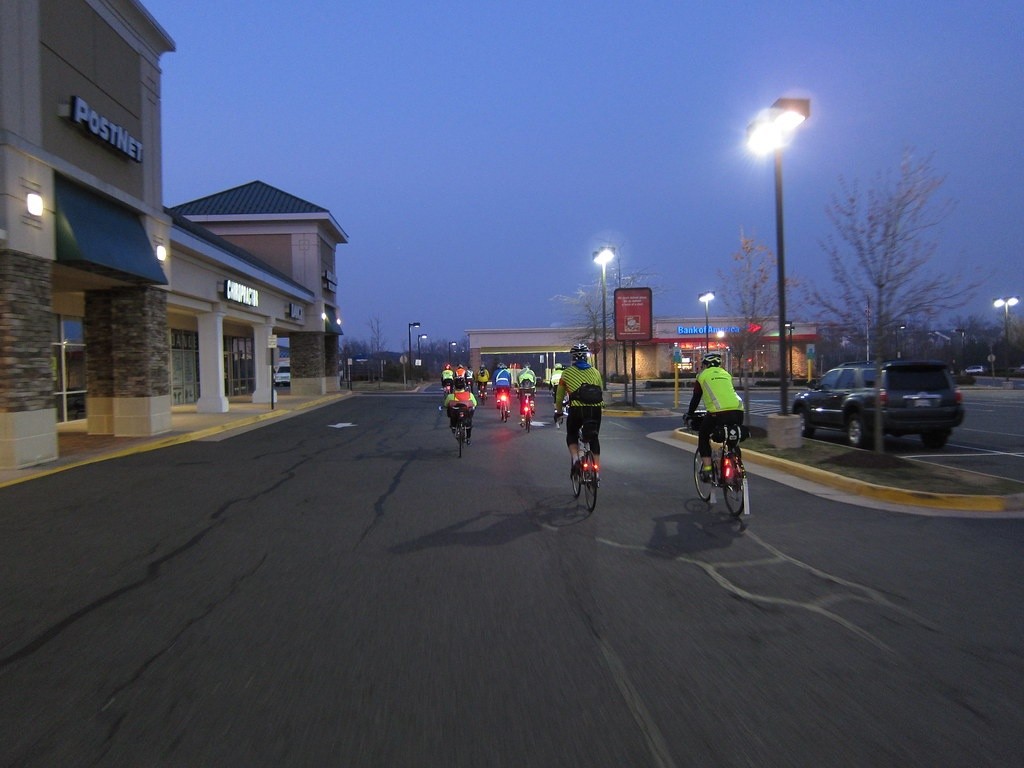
[408,322,420,389]
[418,334,428,388]
[784,319,795,387]
[593,246,615,392]
[744,94,811,415]
[698,291,714,351]
[448,340,457,365]
[992,296,1019,380]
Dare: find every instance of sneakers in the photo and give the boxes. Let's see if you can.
[701,467,714,480]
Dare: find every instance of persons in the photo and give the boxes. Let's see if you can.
[555,343,606,487]
[442,362,569,445]
[681,352,746,491]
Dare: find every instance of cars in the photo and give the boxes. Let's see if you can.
[965,366,985,374]
[792,359,964,449]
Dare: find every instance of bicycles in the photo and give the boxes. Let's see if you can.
[479,389,487,407]
[683,410,749,517]
[520,394,533,430]
[554,410,600,511]
[499,393,508,424]
[455,411,466,458]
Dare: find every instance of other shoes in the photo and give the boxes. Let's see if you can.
[520,417,525,424]
[734,461,747,476]
[571,459,581,473]
[507,407,512,417]
[467,441,472,447]
[496,402,501,410]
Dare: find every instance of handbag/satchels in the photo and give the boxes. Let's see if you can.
[570,384,603,404]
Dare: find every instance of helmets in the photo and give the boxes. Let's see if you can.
[480,364,486,369]
[570,343,589,356]
[555,363,562,370]
[497,362,507,368]
[445,364,452,370]
[457,363,463,368]
[523,363,531,368]
[454,377,464,389]
[701,353,722,366]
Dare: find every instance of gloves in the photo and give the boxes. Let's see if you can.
[554,412,563,425]
